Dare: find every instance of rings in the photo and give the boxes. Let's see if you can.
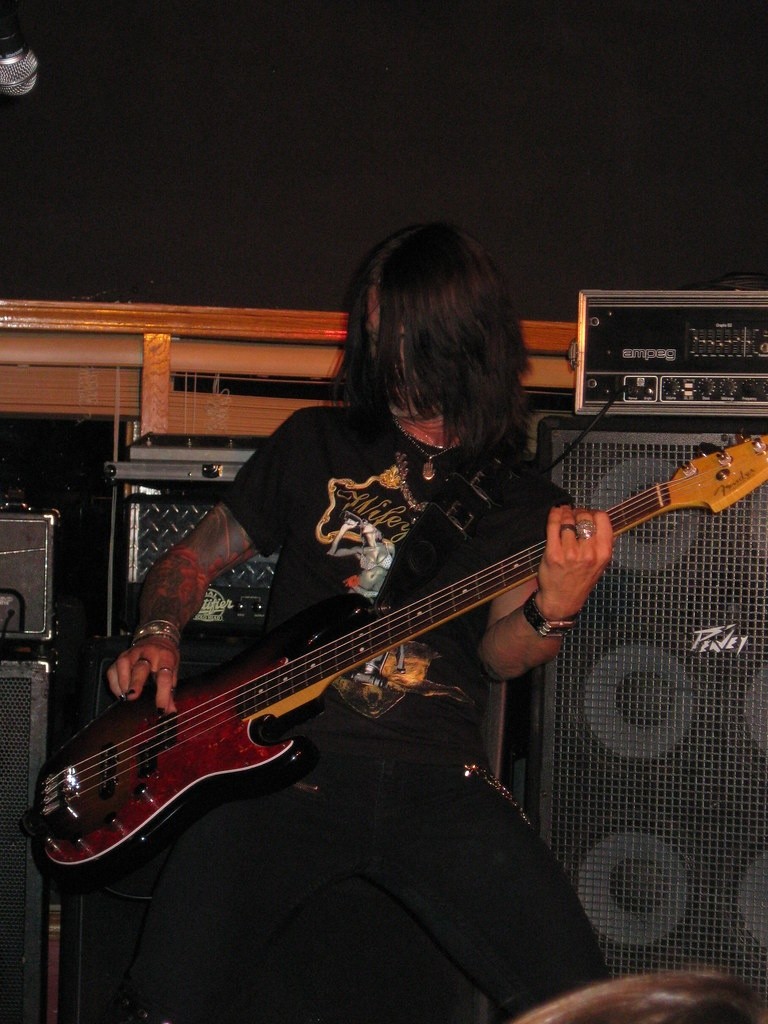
[136,657,152,667]
[156,666,173,675]
[575,520,597,541]
[559,523,576,535]
[130,619,181,649]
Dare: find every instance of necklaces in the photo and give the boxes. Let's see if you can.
[389,414,464,512]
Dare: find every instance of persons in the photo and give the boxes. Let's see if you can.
[100,219,607,1023]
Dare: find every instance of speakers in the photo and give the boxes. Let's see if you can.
[72,638,513,1024]
[0,660,56,1024]
[527,410,768,1011]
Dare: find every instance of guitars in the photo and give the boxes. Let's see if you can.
[31,433,768,886]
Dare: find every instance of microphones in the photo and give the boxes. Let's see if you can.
[0,4,40,97]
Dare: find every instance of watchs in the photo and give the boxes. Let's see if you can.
[522,589,583,640]
